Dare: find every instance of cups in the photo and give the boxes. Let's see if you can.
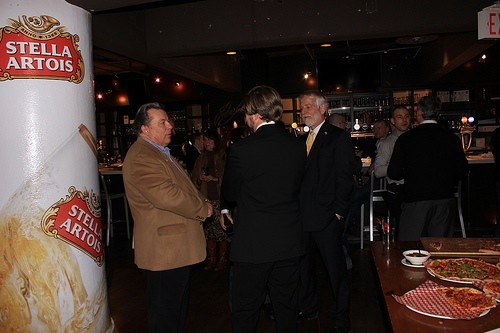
[381,217,394,250]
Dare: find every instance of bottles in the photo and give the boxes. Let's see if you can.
[445,118,462,132]
[452,90,469,102]
[353,109,392,134]
[352,95,389,107]
[436,91,449,102]
[413,108,435,121]
[170,111,186,132]
[223,213,233,236]
[393,97,409,106]
[414,93,428,105]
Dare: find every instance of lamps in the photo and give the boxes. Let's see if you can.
[174,77,186,92]
[112,73,121,89]
[480,51,488,61]
[151,72,162,86]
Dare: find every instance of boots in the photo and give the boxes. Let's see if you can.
[203,239,217,271]
[215,241,230,271]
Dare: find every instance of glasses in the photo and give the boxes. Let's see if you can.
[342,120,349,125]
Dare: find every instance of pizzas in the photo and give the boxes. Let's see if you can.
[427,259,500,282]
[485,282,500,295]
[437,287,496,311]
[478,239,500,255]
[429,241,441,250]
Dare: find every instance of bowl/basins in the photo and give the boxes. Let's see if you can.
[403,248,430,264]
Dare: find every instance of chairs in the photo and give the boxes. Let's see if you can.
[360,170,395,251]
[100,173,130,246]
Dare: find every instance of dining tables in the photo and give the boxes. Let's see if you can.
[369,237,500,333]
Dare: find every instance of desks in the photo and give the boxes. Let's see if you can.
[99,164,125,178]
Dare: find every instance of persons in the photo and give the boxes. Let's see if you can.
[96,86,365,333]
[371,94,500,240]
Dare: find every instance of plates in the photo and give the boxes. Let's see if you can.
[403,286,490,320]
[427,259,495,284]
[483,283,500,303]
[401,258,432,268]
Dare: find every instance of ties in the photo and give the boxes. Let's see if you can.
[306,131,315,157]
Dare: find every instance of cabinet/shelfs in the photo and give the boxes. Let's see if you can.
[322,92,478,131]
